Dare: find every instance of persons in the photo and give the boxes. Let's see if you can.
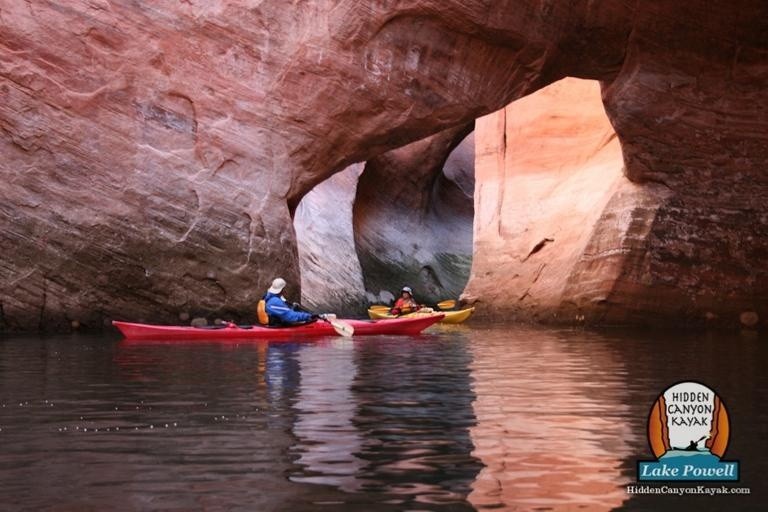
[257,277,323,328]
[390,286,423,316]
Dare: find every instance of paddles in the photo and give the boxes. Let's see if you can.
[370,300,455,313]
[280,295,355,337]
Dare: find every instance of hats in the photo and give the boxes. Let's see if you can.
[401,286,412,296]
[268,278,287,295]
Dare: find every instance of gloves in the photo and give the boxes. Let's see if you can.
[311,314,319,321]
[293,304,299,312]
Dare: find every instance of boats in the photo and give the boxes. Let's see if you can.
[367,303,478,323]
[111,315,339,341]
[290,311,448,336]
